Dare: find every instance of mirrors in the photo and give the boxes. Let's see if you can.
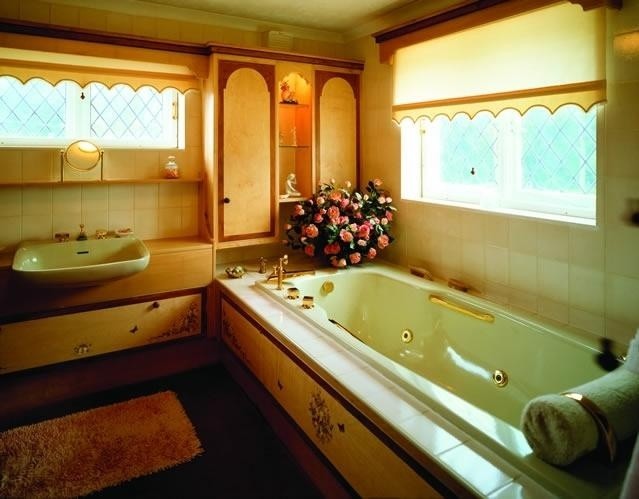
[60,140,104,183]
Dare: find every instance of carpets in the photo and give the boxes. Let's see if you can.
[0,388,205,499]
[0,361,327,498]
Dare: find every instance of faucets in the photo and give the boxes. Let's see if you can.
[276,254,288,291]
[77,225,89,240]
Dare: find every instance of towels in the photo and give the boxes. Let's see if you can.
[519,326,639,467]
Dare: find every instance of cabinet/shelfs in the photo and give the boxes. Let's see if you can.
[202,41,280,252]
[1,287,209,383]
[297,55,364,197]
[277,64,315,242]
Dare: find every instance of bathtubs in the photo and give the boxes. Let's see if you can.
[255,261,629,499]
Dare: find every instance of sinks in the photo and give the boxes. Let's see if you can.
[11,234,150,288]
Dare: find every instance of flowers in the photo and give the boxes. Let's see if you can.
[281,179,398,269]
